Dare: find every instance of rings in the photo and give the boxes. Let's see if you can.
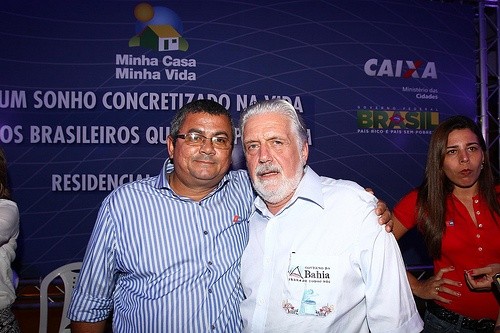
[435,286,440,291]
[388,116,500,333]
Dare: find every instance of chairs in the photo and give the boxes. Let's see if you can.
[38,261,84,333]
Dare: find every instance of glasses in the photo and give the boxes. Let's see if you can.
[175,134,232,150]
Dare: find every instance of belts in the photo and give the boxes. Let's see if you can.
[426,299,496,333]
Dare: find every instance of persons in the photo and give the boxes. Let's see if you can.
[65,100,393,333]
[239,98,424,333]
[0,150,20,333]
